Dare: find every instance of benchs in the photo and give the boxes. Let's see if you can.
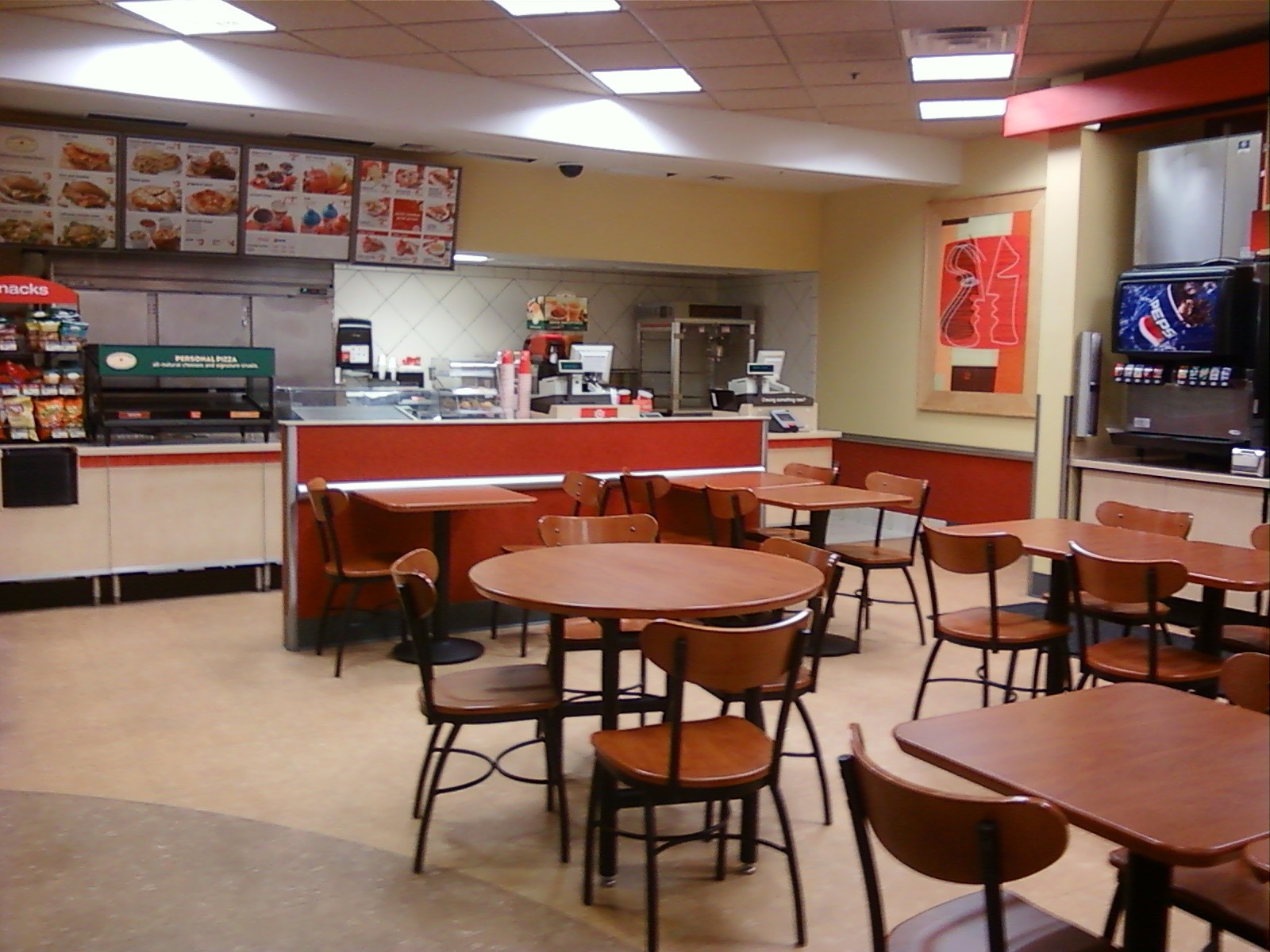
[910,520,1075,721]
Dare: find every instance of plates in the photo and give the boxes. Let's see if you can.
[0,193,22,205]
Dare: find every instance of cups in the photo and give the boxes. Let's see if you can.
[129,230,149,249]
[569,303,581,322]
[618,389,631,404]
[139,219,156,238]
[310,170,327,193]
[637,390,653,400]
[271,200,288,219]
[502,350,514,418]
[517,351,532,418]
[328,165,348,188]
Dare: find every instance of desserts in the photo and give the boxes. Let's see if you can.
[190,151,235,180]
[252,160,293,189]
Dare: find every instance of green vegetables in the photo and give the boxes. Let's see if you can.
[0,218,43,243]
[62,221,106,248]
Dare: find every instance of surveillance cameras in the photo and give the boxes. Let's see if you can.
[558,162,583,178]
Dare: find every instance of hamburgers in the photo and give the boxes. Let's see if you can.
[61,180,105,207]
[1,175,45,203]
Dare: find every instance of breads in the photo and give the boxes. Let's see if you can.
[129,186,176,211]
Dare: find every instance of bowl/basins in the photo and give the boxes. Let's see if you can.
[429,243,445,255]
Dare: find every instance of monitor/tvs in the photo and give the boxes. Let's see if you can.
[570,344,615,395]
[755,349,786,392]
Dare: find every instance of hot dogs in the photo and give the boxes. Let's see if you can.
[61,141,112,172]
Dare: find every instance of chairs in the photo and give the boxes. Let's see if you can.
[1067,538,1224,699]
[490,471,606,659]
[704,481,760,551]
[827,471,933,654]
[1098,654,1270,952]
[616,474,712,546]
[760,462,840,544]
[537,510,669,767]
[695,535,835,844]
[304,476,409,677]
[577,605,809,952]
[390,547,573,876]
[1188,522,1270,657]
[837,721,1118,952]
[1042,500,1194,646]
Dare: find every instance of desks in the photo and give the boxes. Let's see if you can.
[467,541,827,890]
[668,470,824,546]
[891,682,1269,952]
[754,485,914,658]
[937,516,1270,697]
[353,484,537,663]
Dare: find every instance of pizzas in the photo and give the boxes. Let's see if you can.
[190,189,232,214]
